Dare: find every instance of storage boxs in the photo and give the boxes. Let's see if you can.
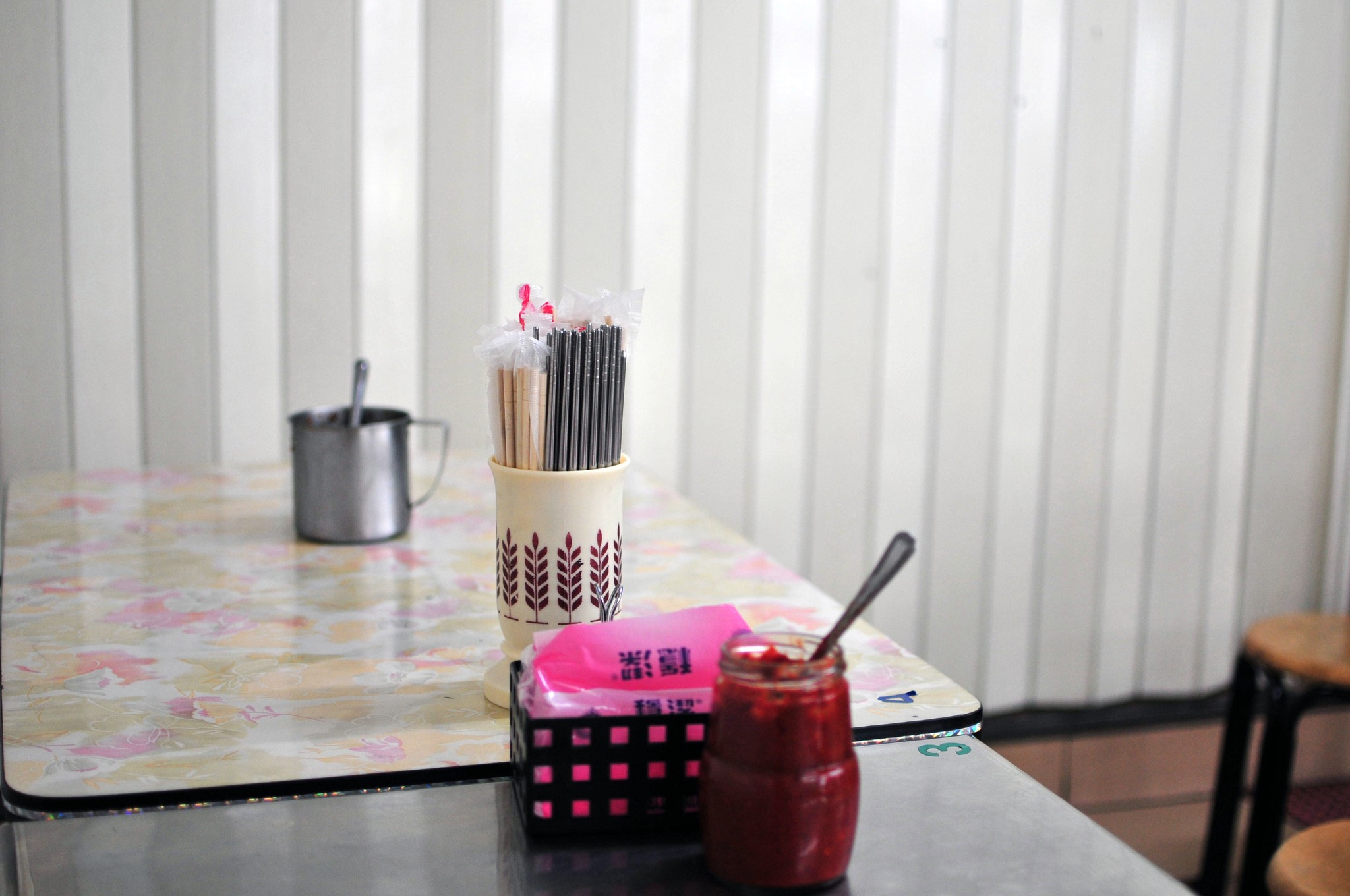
[508,661,716,842]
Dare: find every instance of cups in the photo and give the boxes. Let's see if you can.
[484,453,633,709]
[288,405,451,543]
[699,629,862,893]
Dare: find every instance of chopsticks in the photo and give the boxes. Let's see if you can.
[495,311,626,470]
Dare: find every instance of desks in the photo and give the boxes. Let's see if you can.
[0,733,1206,896]
[0,473,983,812]
[1199,616,1350,896]
